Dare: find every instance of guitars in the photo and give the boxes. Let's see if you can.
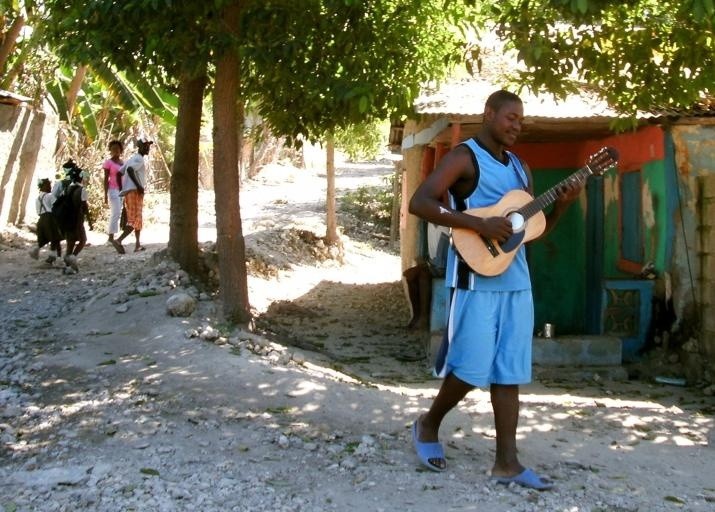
[449,145,618,276]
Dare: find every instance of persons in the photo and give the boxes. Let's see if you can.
[51,160,79,257]
[58,169,93,274]
[414,89,585,490]
[393,201,451,333]
[111,137,152,253]
[29,178,57,265]
[103,140,124,242]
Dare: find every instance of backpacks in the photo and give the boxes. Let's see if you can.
[50,195,78,233]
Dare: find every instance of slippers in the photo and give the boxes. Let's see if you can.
[412,414,448,473]
[490,458,557,491]
[108,237,145,253]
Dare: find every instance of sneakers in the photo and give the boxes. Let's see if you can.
[27,246,80,273]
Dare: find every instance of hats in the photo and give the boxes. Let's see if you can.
[136,137,153,147]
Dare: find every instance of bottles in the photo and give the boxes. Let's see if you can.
[544,321,552,339]
